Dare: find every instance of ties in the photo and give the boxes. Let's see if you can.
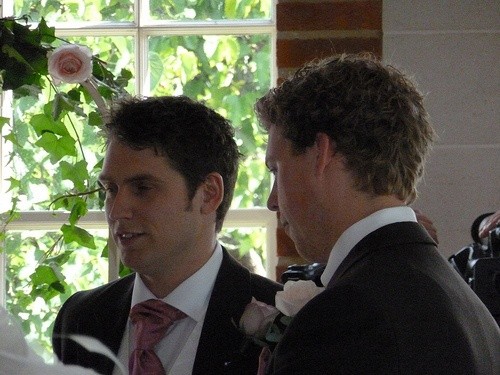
[127,299,189,375]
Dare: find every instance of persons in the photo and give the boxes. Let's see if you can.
[53,95,284,375]
[254,51,500,375]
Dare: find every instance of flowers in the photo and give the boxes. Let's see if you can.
[229,295,279,355]
[266,279,325,355]
[47,43,92,84]
